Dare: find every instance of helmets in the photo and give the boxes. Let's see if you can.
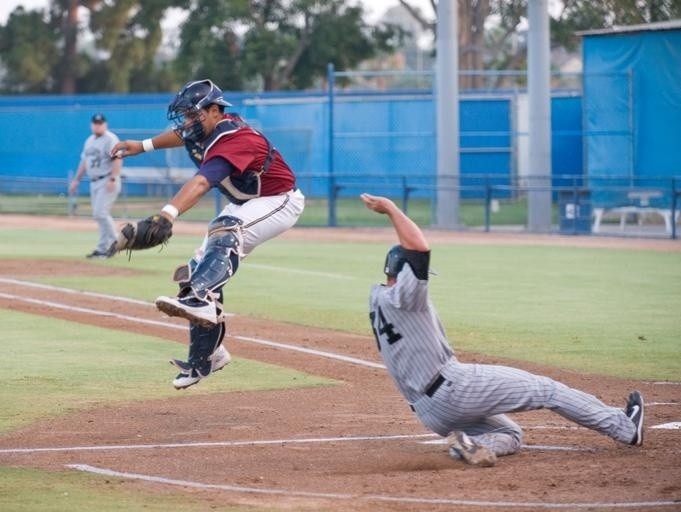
[167,78,233,149]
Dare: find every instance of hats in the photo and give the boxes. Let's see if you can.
[384,245,437,277]
[91,113,105,124]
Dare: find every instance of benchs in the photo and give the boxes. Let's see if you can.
[592,189,681,239]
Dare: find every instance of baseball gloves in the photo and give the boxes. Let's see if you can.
[105,213,172,261]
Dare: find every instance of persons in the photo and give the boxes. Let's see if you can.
[67,112,125,259]
[109,76,307,391]
[356,191,645,470]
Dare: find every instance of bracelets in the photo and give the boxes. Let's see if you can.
[159,204,179,220]
[141,137,154,153]
[109,177,116,182]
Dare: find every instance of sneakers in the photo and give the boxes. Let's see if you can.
[85,241,118,260]
[623,390,645,447]
[446,429,498,468]
[172,342,232,390]
[155,293,218,330]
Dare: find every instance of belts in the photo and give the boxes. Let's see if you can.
[410,374,445,412]
